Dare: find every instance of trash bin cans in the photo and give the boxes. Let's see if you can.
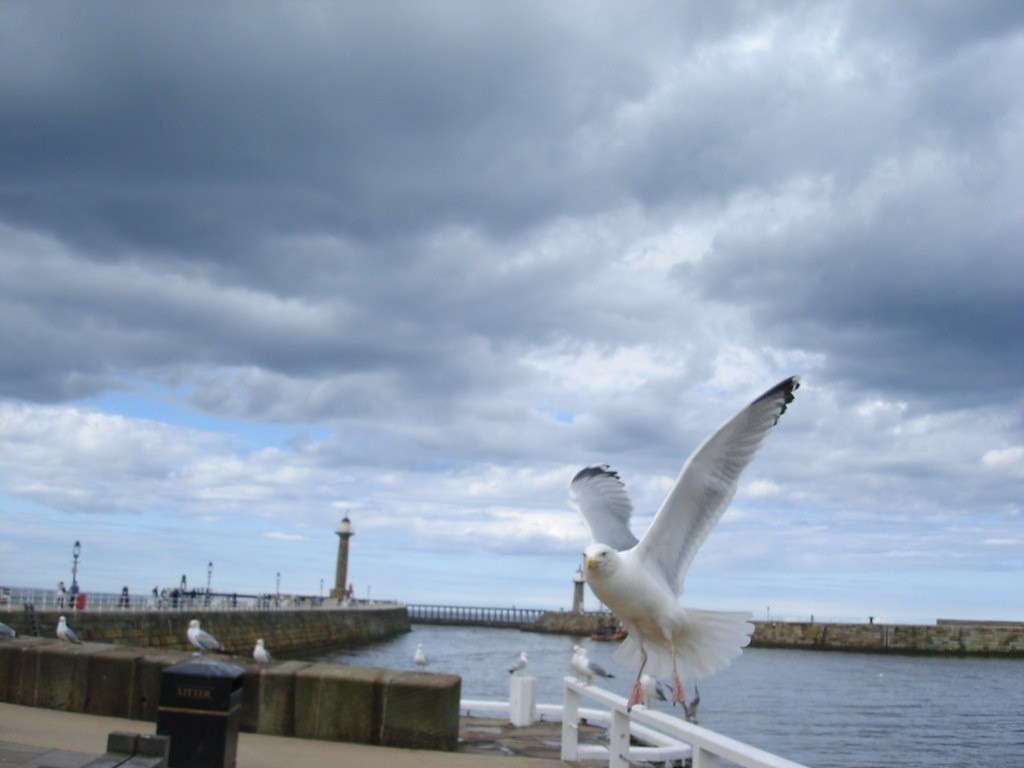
[155,661,247,768]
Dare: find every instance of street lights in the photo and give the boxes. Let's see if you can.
[69,540,81,609]
[205,560,214,609]
[275,572,282,607]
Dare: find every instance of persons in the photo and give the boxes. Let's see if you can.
[117,587,130,607]
[54,581,79,608]
[149,587,280,608]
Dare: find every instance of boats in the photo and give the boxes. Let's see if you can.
[592,612,623,642]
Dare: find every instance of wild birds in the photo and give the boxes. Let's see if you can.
[0,622,18,639]
[187,620,226,656]
[56,616,81,645]
[508,652,528,674]
[253,639,270,663]
[640,676,666,707]
[570,376,800,716]
[570,645,616,686]
[414,643,429,666]
